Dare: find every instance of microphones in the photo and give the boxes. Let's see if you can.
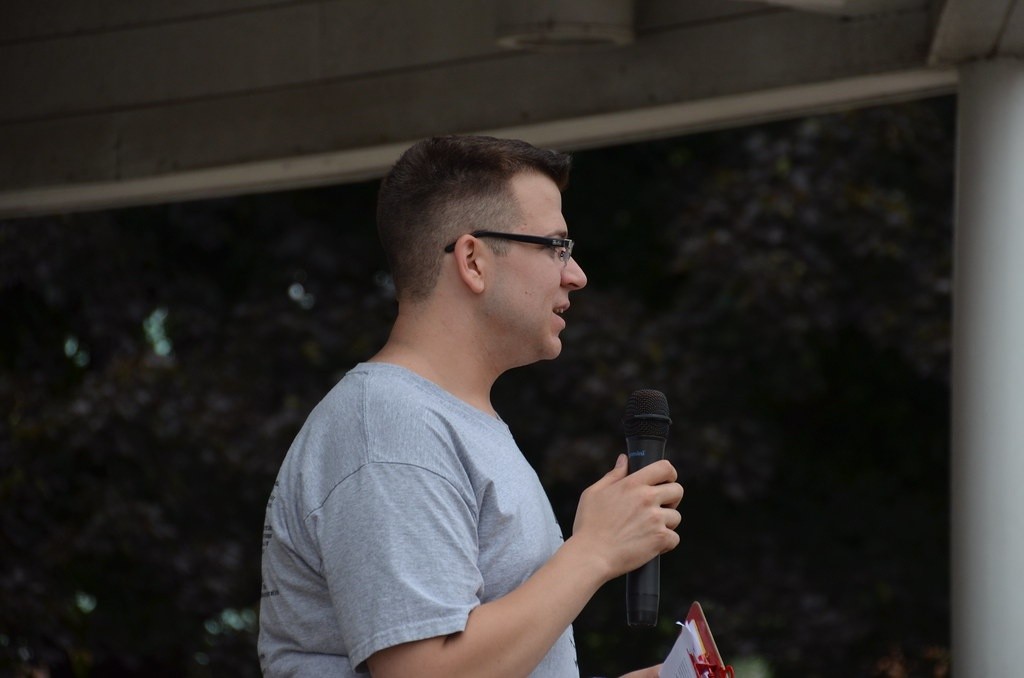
[623,388,672,627]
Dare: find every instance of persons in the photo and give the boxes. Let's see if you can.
[256,134,685,677]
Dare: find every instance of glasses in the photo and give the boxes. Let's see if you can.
[441,229,573,258]
